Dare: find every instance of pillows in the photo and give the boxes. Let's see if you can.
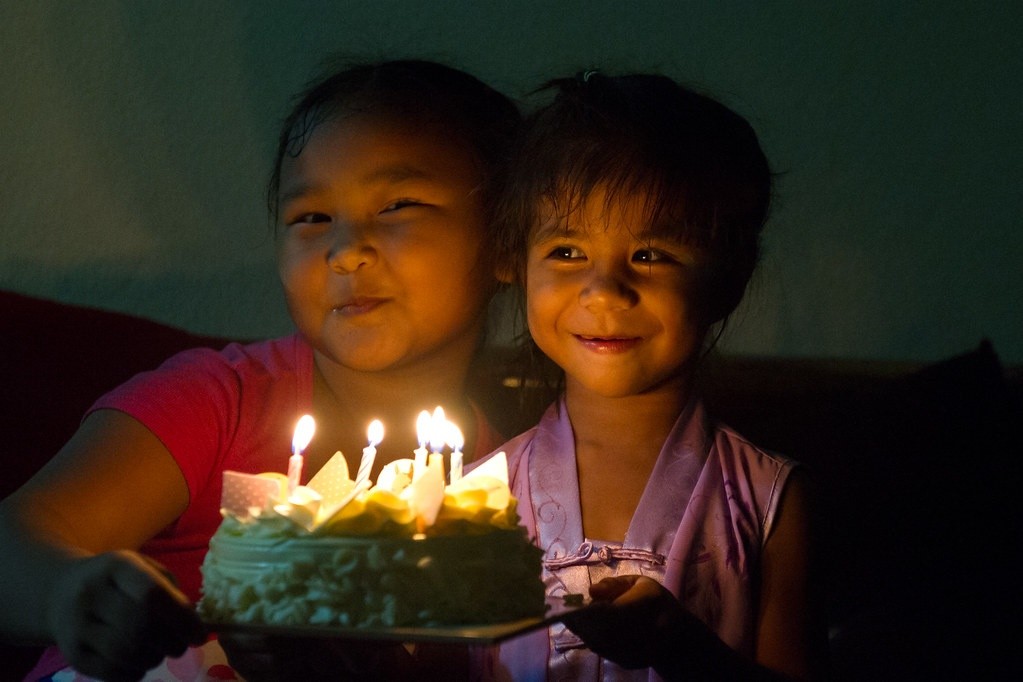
[730,337,1023,682]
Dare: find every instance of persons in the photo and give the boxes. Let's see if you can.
[0,61,522,682]
[448,67,809,682]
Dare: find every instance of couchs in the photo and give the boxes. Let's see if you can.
[0,288,1023,682]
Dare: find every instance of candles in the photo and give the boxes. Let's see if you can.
[287,405,465,491]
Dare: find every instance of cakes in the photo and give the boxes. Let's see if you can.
[197,450,552,629]
